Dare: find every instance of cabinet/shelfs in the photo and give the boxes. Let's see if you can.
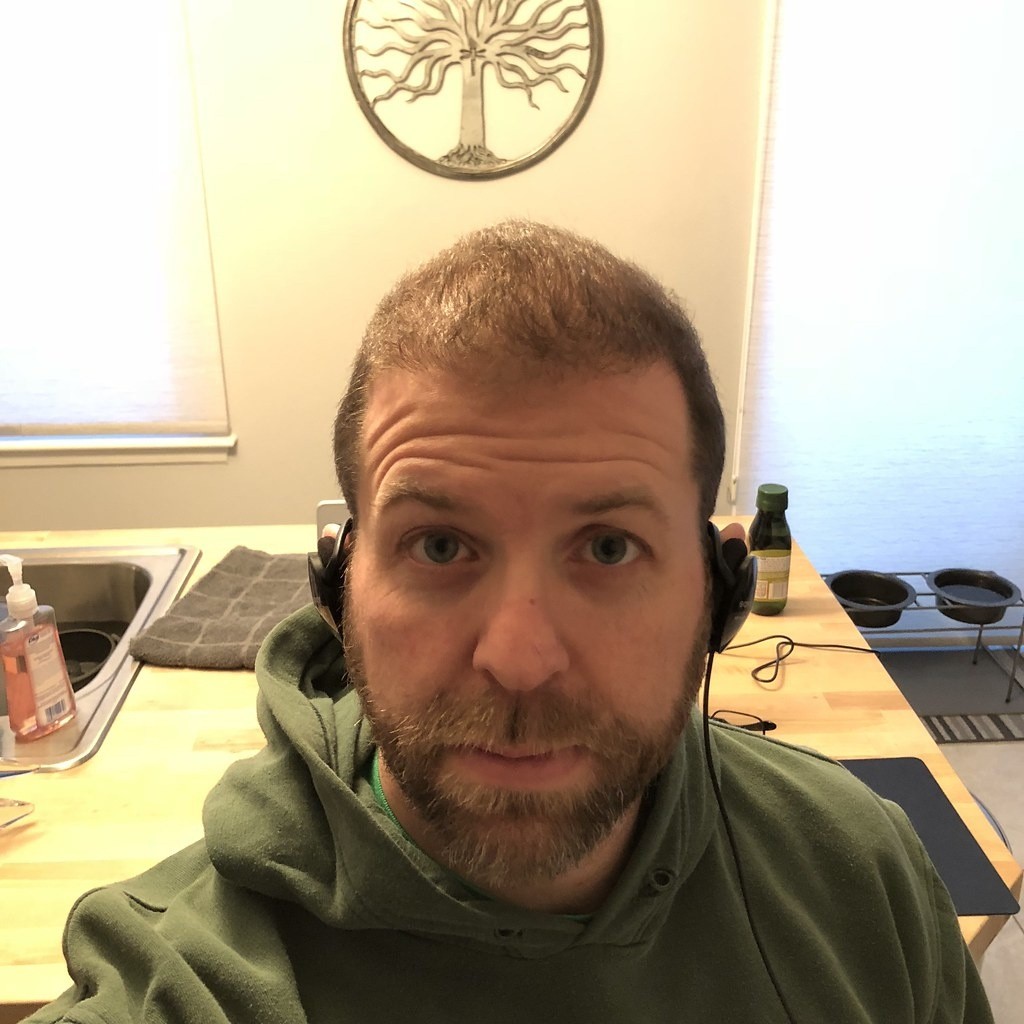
[820,567,1024,705]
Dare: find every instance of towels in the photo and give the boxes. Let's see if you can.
[129,542,330,670]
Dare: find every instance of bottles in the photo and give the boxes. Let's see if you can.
[748,484,792,616]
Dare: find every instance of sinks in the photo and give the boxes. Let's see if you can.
[0,540,202,771]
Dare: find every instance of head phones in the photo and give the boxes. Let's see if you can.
[309,514,760,657]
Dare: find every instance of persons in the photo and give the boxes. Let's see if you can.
[13,220,997,1024]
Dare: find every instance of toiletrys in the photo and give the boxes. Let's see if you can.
[0,551,79,744]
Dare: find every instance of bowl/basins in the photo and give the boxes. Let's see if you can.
[825,568,918,629]
[926,568,1021,624]
